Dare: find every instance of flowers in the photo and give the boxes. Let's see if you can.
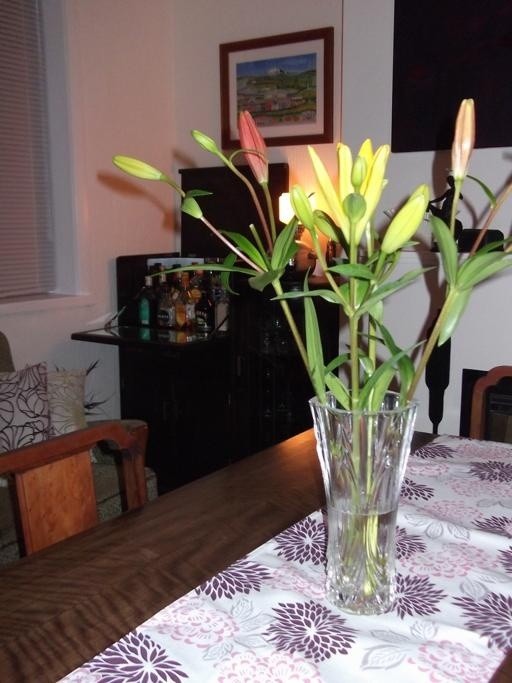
[107,85,512,601]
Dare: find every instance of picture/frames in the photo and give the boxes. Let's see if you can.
[219,22,333,151]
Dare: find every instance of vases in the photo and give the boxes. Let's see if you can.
[308,385,422,611]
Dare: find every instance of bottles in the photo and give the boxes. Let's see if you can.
[139,259,234,333]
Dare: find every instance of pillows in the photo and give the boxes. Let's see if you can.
[0,361,54,490]
[45,365,97,462]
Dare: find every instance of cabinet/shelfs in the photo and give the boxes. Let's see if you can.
[236,278,342,457]
[67,248,277,481]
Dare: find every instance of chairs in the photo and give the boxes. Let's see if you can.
[1,409,145,559]
[1,329,157,568]
[469,362,510,447]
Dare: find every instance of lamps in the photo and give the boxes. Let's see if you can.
[265,185,320,279]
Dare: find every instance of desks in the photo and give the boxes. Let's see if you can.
[3,407,512,682]
